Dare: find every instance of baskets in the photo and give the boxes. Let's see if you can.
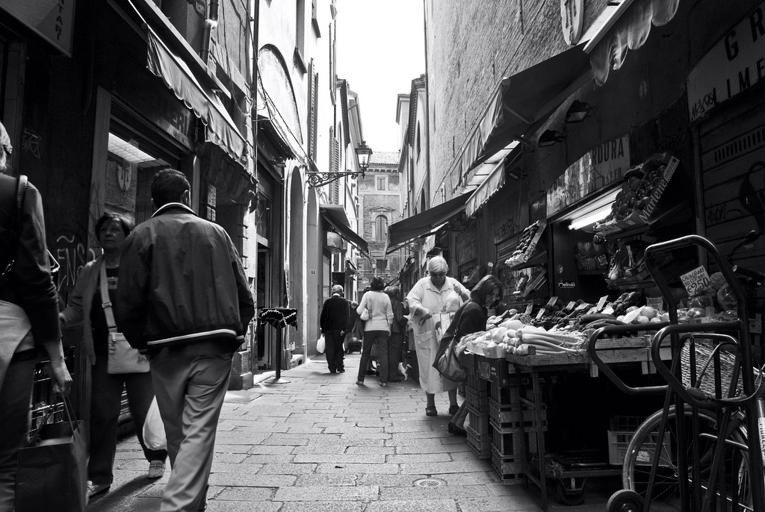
[607,412,673,467]
[681,337,764,399]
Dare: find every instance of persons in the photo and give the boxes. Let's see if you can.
[406,256,471,415]
[1,116,62,512]
[114,168,254,511]
[433,274,503,435]
[60,212,166,499]
[320,276,408,387]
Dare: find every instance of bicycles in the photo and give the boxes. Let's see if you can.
[620,260,765,511]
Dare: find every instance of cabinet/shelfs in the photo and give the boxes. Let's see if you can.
[26,352,79,444]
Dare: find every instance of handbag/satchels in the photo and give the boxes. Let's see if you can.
[433,311,455,343]
[15,388,91,510]
[106,332,150,373]
[438,339,468,381]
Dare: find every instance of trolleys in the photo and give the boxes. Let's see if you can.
[587,230,765,512]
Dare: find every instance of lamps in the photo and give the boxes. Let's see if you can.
[306,141,372,190]
[568,197,649,231]
[355,258,377,272]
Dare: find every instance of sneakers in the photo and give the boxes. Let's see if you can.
[426,406,465,434]
[149,460,164,477]
[86,478,110,495]
